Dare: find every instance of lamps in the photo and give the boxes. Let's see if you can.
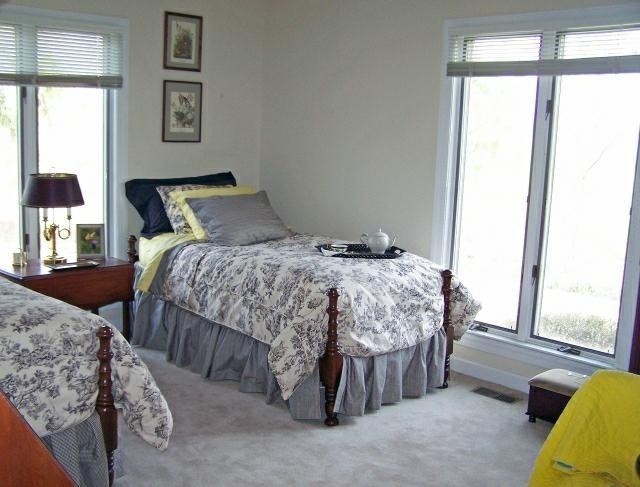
[24,171,85,263]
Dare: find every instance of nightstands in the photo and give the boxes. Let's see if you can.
[1,257,135,343]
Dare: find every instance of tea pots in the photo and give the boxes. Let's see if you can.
[359,228,400,255]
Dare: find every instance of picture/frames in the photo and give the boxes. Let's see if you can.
[163,79,203,142]
[162,9,204,73]
[75,224,106,260]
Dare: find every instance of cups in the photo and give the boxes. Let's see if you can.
[13,252,28,265]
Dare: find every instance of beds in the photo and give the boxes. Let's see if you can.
[126,230,482,427]
[0,275,174,486]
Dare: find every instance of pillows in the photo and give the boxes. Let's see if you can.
[124,171,294,246]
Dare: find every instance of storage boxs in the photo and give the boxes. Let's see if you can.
[525,367,591,426]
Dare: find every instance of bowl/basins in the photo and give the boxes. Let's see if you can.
[330,244,348,253]
